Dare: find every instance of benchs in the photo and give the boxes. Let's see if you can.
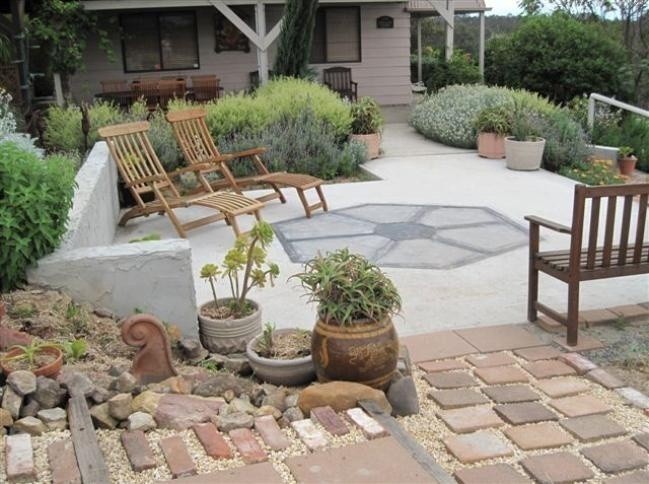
[525,180,648,349]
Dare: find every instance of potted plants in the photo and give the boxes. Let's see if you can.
[247,325,316,386]
[2,335,65,379]
[347,94,385,159]
[199,218,281,356]
[617,146,638,175]
[504,108,547,172]
[473,104,510,159]
[287,247,404,391]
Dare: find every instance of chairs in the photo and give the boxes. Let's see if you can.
[248,68,276,92]
[322,64,359,101]
[93,73,227,112]
[100,119,264,245]
[163,108,329,217]
